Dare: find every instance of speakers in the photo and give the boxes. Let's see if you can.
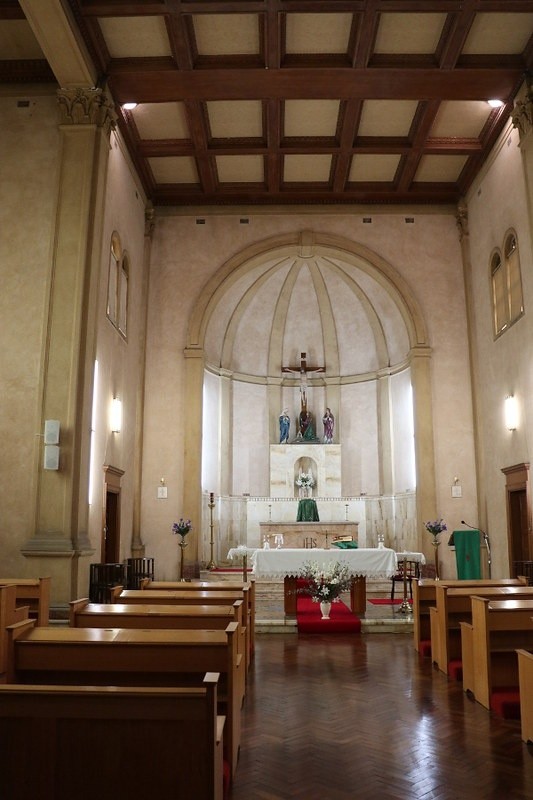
[44,445,60,470]
[44,419,60,445]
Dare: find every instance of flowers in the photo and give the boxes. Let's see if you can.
[171,517,194,542]
[423,518,447,541]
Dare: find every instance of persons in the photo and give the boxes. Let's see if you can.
[285,367,323,406]
[301,412,318,441]
[277,409,291,444]
[322,408,336,443]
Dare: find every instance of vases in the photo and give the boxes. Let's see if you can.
[431,542,442,581]
[177,543,188,582]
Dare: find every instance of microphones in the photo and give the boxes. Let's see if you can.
[461,521,475,529]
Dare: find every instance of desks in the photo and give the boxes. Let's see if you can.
[515,648,532,748]
[460,596,532,711]
[409,574,527,657]
[250,548,398,618]
[0,574,255,799]
[429,584,533,678]
